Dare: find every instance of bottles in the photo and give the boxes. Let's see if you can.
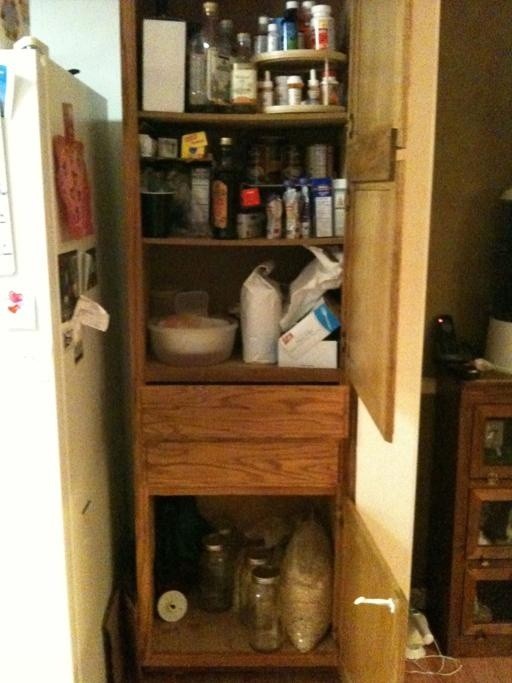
[185,0,346,112]
[211,136,237,238]
[192,521,286,650]
[432,310,456,360]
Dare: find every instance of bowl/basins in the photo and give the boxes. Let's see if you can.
[141,186,174,236]
[143,306,238,368]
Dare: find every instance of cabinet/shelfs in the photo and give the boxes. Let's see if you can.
[446,374,512,661]
[115,0,444,682]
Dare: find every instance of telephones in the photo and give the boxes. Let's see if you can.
[435,314,476,374]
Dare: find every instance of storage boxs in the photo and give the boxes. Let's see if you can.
[137,15,186,115]
